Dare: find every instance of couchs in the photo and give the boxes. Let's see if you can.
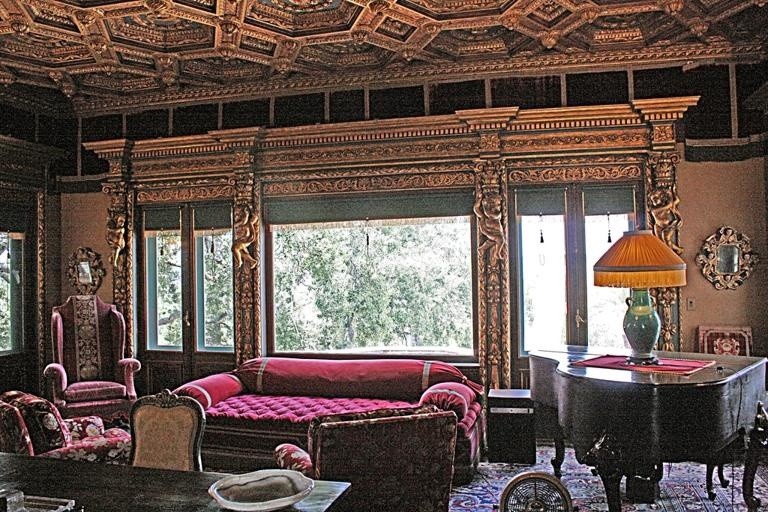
[0,392,131,467]
[273,403,458,511]
[172,358,483,485]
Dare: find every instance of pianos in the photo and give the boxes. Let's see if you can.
[529,346,768,512]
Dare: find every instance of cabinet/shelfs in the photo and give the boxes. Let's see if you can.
[488,389,536,465]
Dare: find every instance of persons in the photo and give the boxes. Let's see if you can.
[102,214,127,268]
[650,189,685,256]
[473,191,505,260]
[232,204,259,270]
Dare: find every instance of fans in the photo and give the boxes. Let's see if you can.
[501,470,573,511]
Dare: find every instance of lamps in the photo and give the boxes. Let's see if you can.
[595,230,687,364]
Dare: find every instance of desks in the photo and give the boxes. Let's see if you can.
[0,453,352,511]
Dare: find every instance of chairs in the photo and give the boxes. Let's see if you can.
[43,296,141,421]
[131,388,206,471]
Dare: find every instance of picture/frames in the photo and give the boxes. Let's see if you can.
[698,326,754,357]
